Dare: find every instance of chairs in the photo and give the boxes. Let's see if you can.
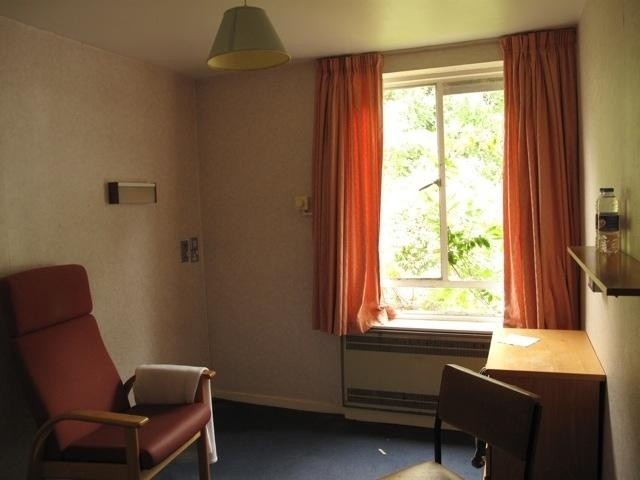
[370,359,544,480]
[0,264,222,479]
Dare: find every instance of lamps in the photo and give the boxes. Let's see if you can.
[206,1,291,73]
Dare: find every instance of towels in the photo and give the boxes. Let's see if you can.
[133,364,218,466]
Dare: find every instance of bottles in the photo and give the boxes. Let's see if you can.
[595,188,620,255]
